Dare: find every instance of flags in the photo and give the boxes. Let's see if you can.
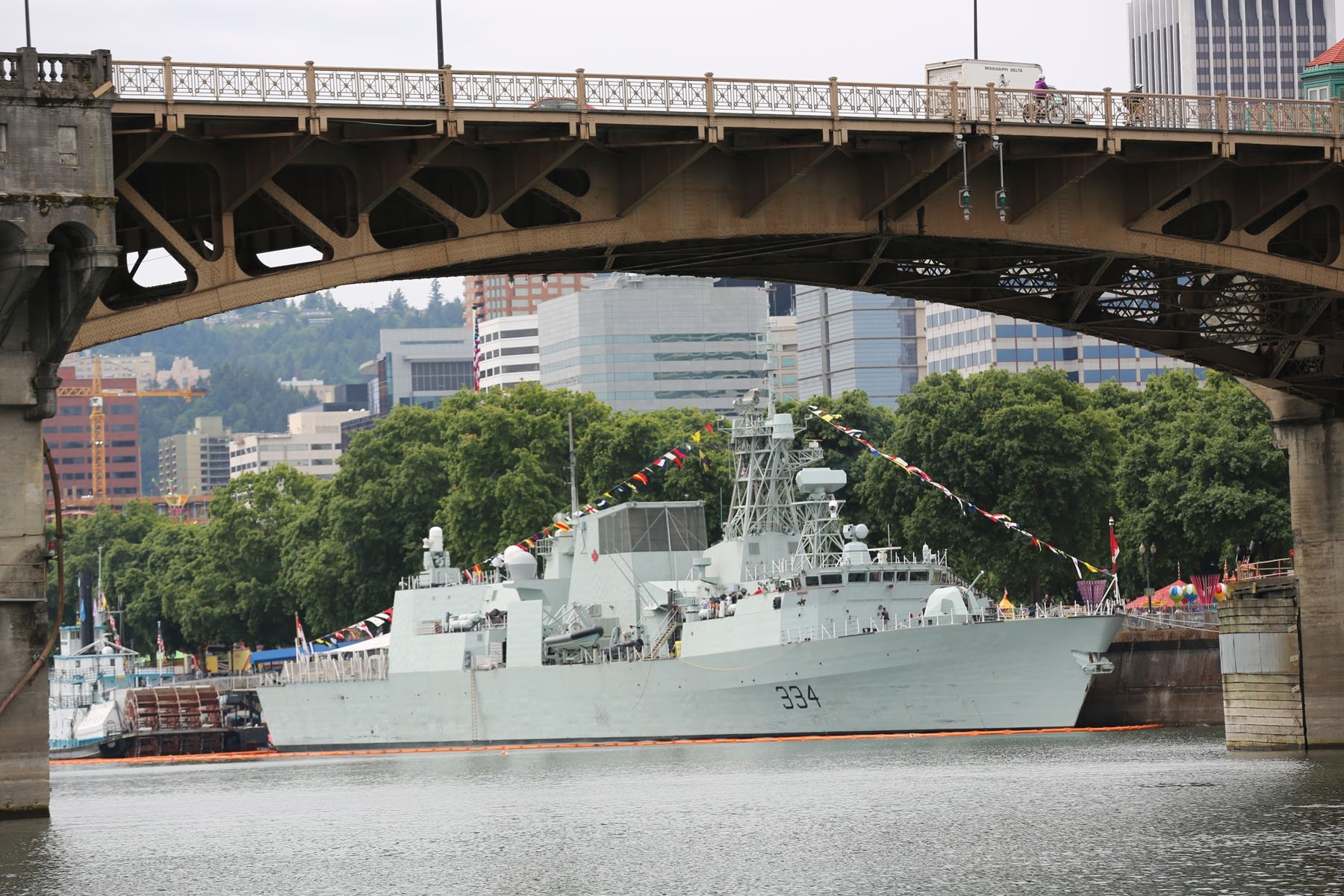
[100,595,125,653]
[92,599,98,618]
[808,404,1110,578]
[308,416,714,658]
[80,599,87,623]
[296,616,308,655]
[157,626,168,664]
[1110,527,1121,574]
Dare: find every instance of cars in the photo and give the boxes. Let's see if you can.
[528,97,597,110]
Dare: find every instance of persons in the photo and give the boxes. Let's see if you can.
[1042,594,1055,608]
[1033,74,1055,123]
[707,578,802,610]
[1122,83,1151,121]
[881,607,889,630]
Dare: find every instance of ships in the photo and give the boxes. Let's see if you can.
[254,314,1125,750]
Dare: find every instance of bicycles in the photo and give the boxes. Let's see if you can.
[1022,95,1065,124]
[1115,105,1151,127]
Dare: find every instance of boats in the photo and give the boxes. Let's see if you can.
[47,545,178,760]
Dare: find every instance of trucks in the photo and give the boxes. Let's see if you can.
[924,58,1068,124]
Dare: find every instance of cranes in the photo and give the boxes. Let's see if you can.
[55,356,207,509]
